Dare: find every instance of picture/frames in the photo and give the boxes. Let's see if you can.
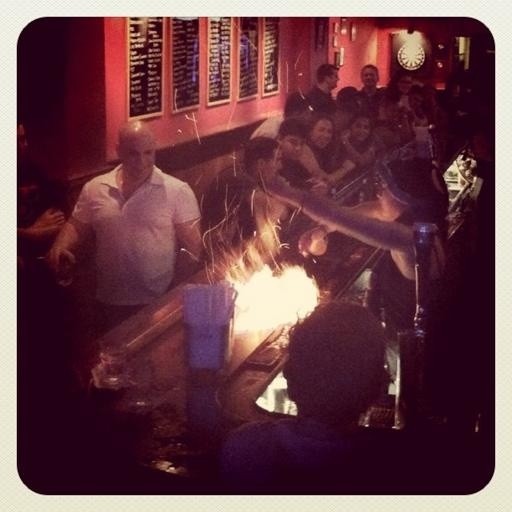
[333,22,338,33]
[334,52,340,69]
[350,22,357,43]
[340,17,348,36]
[332,36,337,47]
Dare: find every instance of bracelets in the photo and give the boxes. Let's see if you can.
[297,193,307,215]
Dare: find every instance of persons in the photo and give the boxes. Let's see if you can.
[251,63,471,193]
[18,118,63,276]
[47,119,202,334]
[227,301,392,471]
[203,136,278,242]
[256,157,448,342]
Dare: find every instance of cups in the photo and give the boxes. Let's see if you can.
[181,324,233,369]
[183,283,239,326]
